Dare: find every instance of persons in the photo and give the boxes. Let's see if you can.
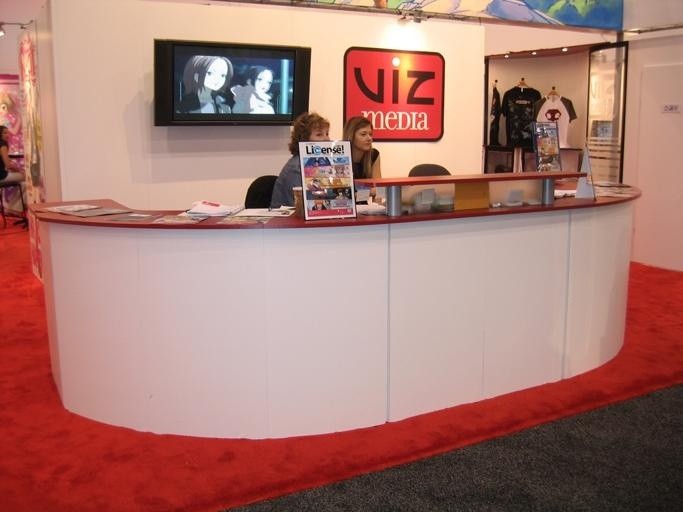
[269,111,332,211]
[342,116,382,205]
[0,126,25,216]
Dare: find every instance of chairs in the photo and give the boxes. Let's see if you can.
[243,173,278,210]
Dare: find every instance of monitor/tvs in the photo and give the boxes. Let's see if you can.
[154,39,311,126]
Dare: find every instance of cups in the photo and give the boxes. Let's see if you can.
[292,187,304,218]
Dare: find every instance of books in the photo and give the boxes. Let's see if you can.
[183,198,245,217]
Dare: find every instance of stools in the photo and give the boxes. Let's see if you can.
[0,182,23,227]
